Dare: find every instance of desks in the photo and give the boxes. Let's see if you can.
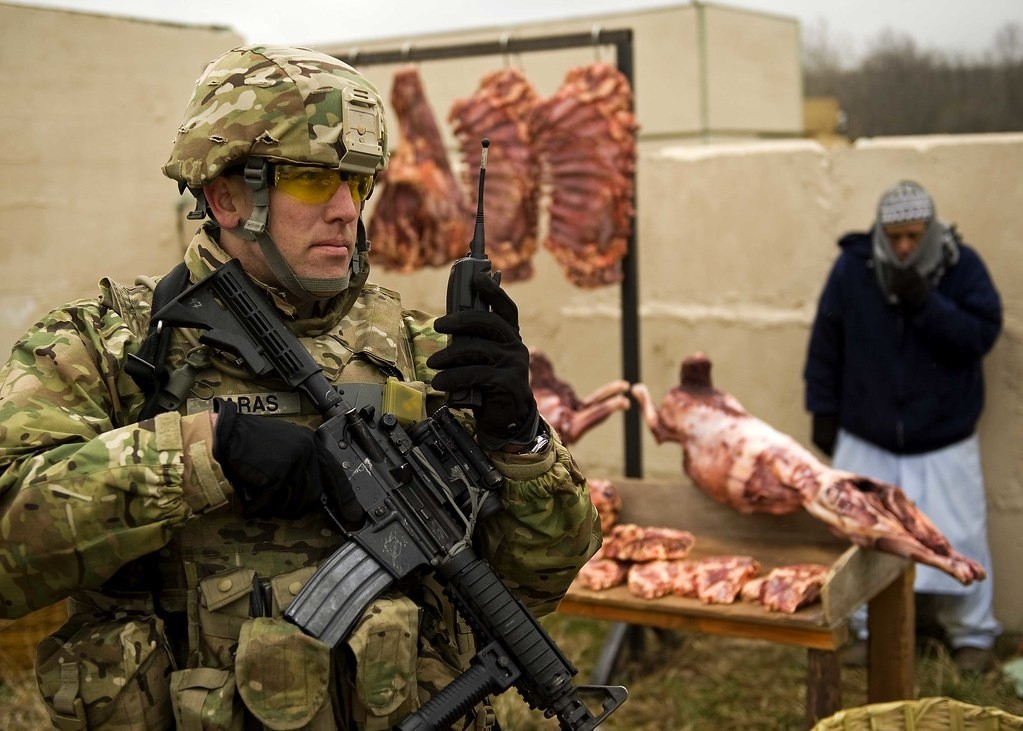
[554,475,917,731]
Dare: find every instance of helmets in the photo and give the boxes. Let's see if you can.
[161,44,390,189]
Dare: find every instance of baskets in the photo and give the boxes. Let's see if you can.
[809,696,1023,731]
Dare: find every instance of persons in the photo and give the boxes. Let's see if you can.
[801,179,1006,673]
[0,42,604,731]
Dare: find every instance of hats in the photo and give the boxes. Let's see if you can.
[879,184,932,223]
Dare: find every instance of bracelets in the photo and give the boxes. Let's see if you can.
[525,433,550,453]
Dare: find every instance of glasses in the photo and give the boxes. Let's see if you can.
[266,164,375,205]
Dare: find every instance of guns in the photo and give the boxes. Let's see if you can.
[147,257,630,730]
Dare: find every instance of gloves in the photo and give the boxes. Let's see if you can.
[211,397,355,520]
[426,271,541,450]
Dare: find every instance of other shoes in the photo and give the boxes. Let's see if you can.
[841,641,866,668]
[955,647,986,678]
